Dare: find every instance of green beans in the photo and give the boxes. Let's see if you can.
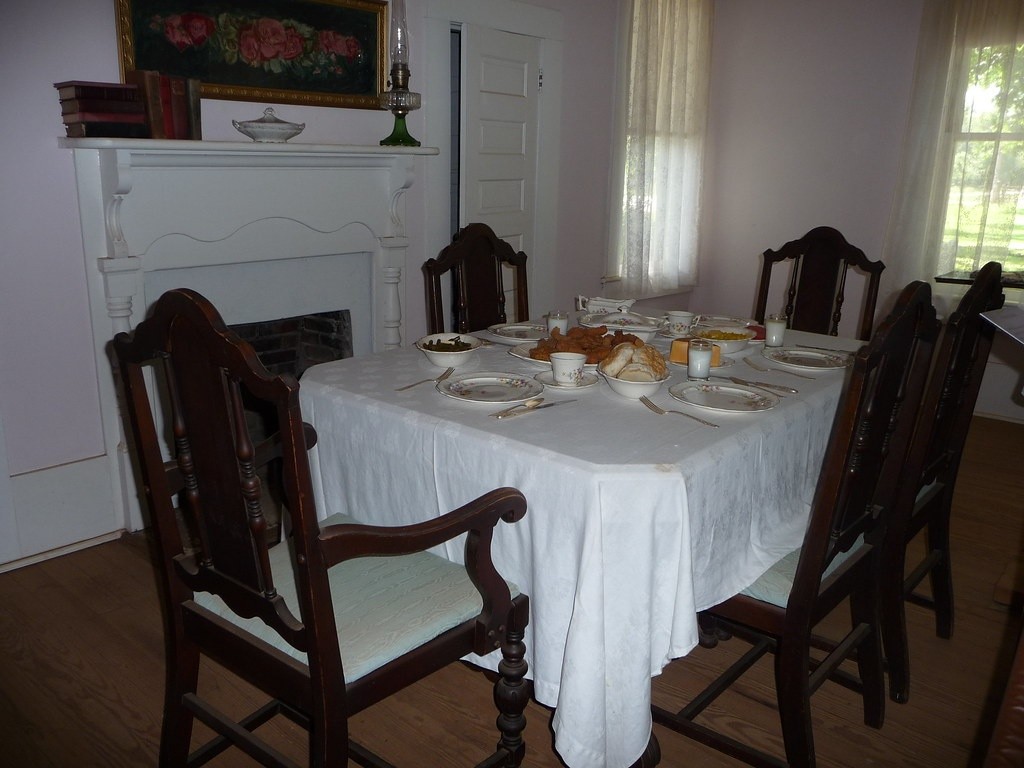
[423,339,473,352]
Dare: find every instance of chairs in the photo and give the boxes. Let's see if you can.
[652,281,941,767]
[882,263,1005,703]
[754,226,886,342]
[422,222,529,334]
[104,287,528,768]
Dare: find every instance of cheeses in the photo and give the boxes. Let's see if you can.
[669,337,721,367]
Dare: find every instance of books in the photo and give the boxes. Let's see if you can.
[144,70,202,140]
[53,80,151,138]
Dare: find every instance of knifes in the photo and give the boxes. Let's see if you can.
[496,399,577,419]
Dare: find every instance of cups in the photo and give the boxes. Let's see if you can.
[547,310,569,336]
[550,352,587,386]
[687,338,713,381]
[765,313,787,348]
[662,310,694,337]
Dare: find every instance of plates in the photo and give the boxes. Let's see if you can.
[740,325,766,344]
[508,341,598,368]
[697,314,759,328]
[669,381,780,414]
[665,352,735,369]
[487,323,548,340]
[534,371,599,390]
[761,347,854,370]
[436,372,544,403]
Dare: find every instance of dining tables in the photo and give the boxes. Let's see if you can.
[298,307,868,768]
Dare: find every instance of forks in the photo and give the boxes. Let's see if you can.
[395,366,455,392]
[638,394,720,429]
[743,357,816,381]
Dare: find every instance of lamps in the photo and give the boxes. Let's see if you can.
[380,0,421,147]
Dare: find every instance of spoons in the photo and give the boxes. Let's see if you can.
[487,398,544,418]
[730,376,784,398]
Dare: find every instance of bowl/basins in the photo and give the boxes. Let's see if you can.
[690,326,757,354]
[414,332,483,367]
[579,306,666,343]
[232,107,307,142]
[597,365,671,400]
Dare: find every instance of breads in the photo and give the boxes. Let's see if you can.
[598,342,668,383]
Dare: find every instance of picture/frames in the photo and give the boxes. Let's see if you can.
[115,0,388,111]
